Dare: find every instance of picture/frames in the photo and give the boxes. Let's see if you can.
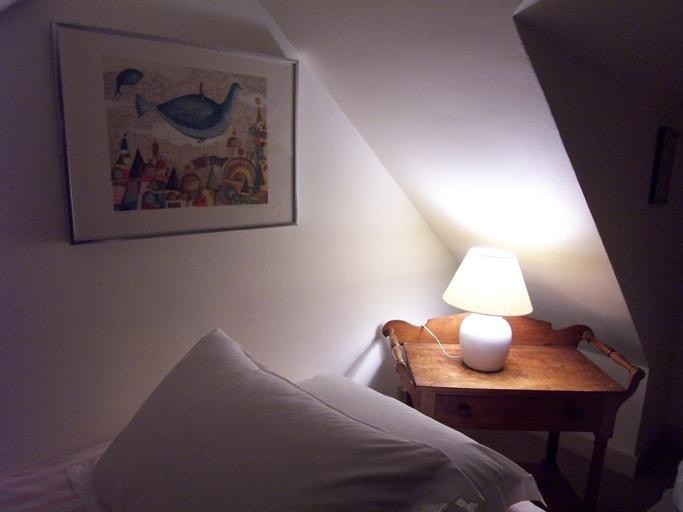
[50,18,300,247]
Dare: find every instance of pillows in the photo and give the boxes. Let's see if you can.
[60,326,484,511]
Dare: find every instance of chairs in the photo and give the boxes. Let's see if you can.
[290,372,546,512]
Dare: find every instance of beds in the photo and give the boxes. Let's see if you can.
[0,374,545,512]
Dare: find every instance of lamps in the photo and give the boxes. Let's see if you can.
[440,245,534,373]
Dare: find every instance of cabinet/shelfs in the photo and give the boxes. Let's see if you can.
[380,313,646,511]
